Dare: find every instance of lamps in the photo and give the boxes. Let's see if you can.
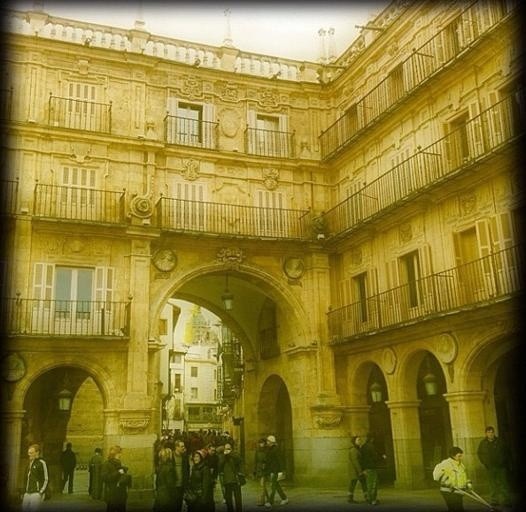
[370,366,382,402]
[57,367,73,410]
[423,352,438,396]
[221,270,234,311]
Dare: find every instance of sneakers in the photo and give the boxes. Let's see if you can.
[256,496,288,508]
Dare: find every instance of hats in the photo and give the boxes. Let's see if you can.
[267,435,279,447]
[224,444,232,455]
[196,448,208,459]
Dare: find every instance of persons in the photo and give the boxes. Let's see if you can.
[346,436,372,504]
[87,427,247,511]
[60,440,77,495]
[476,425,512,505]
[360,434,389,504]
[18,444,52,510]
[431,447,474,510]
[263,435,290,508]
[250,437,273,507]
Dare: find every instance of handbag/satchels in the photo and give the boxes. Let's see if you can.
[237,473,247,487]
[44,487,52,501]
